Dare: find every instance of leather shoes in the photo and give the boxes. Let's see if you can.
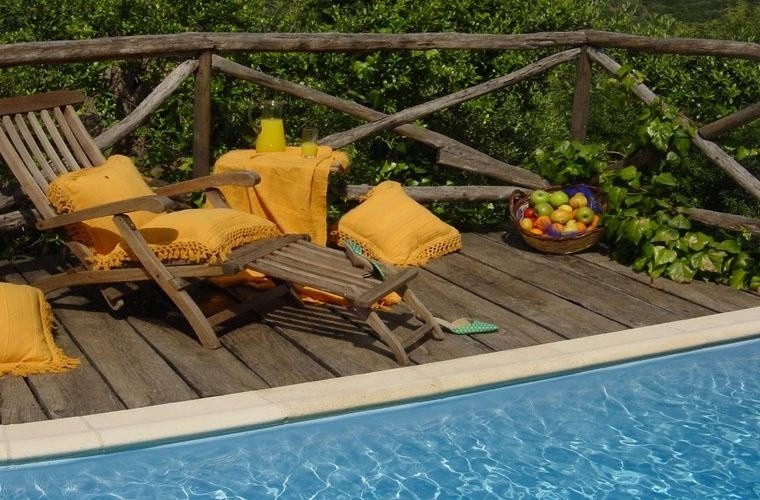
[416,314,498,335]
[342,239,398,282]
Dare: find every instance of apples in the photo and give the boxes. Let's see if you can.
[521,190,594,235]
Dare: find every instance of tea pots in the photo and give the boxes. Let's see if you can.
[250,99,289,154]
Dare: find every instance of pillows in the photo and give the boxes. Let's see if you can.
[328,178,461,267]
[0,282,80,376]
[48,152,278,261]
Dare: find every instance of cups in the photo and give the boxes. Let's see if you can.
[301,128,317,158]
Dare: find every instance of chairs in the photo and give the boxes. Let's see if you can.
[0,90,443,367]
